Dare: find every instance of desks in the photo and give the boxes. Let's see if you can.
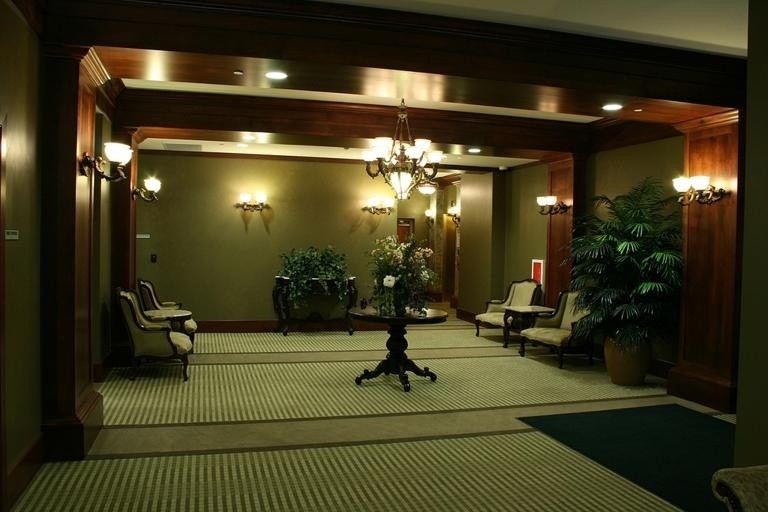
[344,307,448,391]
[271,276,360,335]
[503,306,556,355]
[142,309,191,333]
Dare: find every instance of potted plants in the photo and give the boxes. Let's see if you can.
[559,175,696,384]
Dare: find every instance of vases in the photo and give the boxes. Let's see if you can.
[394,283,405,316]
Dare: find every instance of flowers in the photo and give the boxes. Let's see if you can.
[363,233,434,315]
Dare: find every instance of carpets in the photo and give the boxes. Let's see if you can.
[512,402,734,510]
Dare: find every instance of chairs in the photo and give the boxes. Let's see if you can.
[713,462,767,510]
[476,278,541,348]
[518,288,596,369]
[115,287,192,381]
[138,279,197,353]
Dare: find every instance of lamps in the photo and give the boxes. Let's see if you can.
[670,175,731,206]
[361,196,395,216]
[448,206,460,222]
[131,177,161,202]
[363,98,443,200]
[535,196,571,215]
[416,182,439,197]
[424,209,434,223]
[81,141,133,182]
[235,192,271,211]
